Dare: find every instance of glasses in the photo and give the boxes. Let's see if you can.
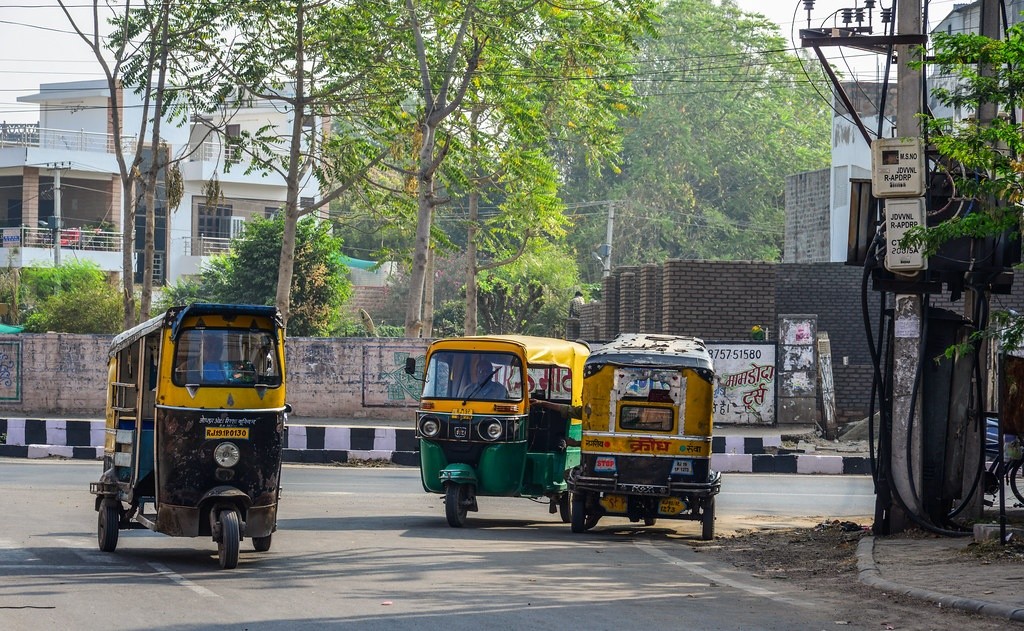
[478,369,492,375]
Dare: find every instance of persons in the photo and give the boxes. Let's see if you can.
[456,355,510,401]
[175,333,232,384]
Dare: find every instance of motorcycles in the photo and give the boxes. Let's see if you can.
[88,302,293,567]
[563,333,728,536]
[406,333,595,524]
